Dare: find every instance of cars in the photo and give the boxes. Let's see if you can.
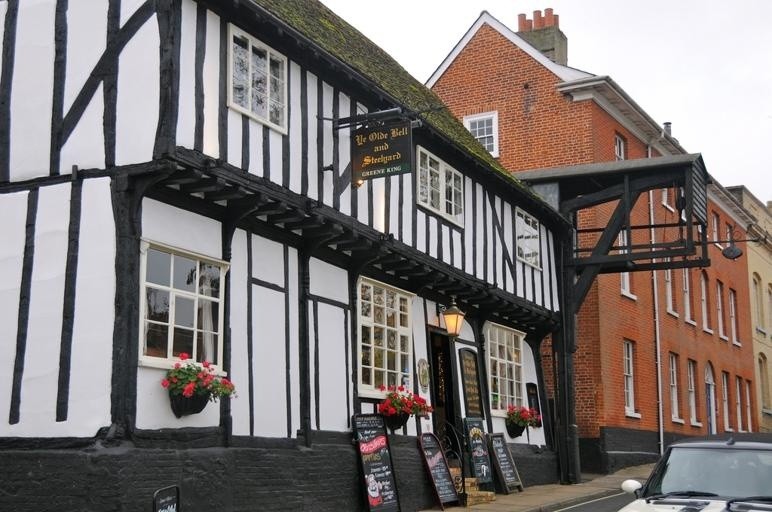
[617,432,772,512]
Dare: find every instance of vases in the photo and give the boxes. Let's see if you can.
[507,420,525,438]
[385,414,409,431]
[168,390,207,419]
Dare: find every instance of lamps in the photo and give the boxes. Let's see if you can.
[437,292,467,344]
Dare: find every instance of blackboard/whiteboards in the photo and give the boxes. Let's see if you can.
[419,433,458,504]
[489,433,521,486]
[352,413,399,511]
[466,418,492,483]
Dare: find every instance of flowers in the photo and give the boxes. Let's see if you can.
[161,352,240,403]
[506,405,543,430]
[379,382,435,420]
